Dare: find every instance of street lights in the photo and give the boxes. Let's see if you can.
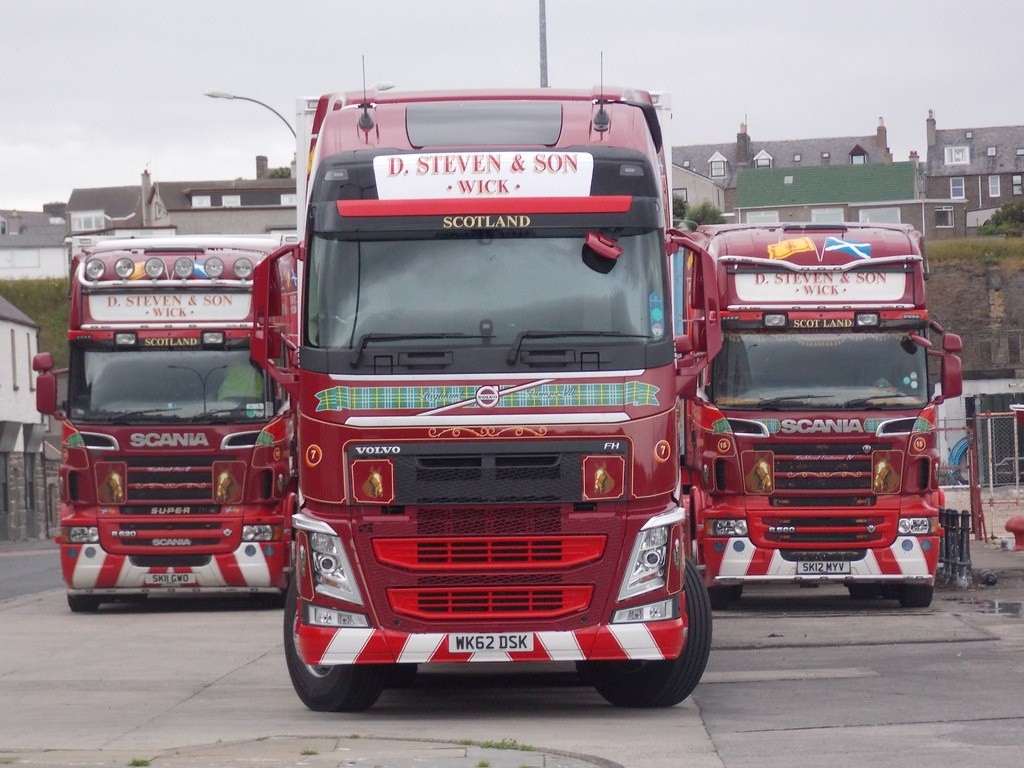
[202,88,297,145]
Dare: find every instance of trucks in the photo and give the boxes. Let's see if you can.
[245,80,721,712]
[672,220,964,609]
[29,233,298,608]
